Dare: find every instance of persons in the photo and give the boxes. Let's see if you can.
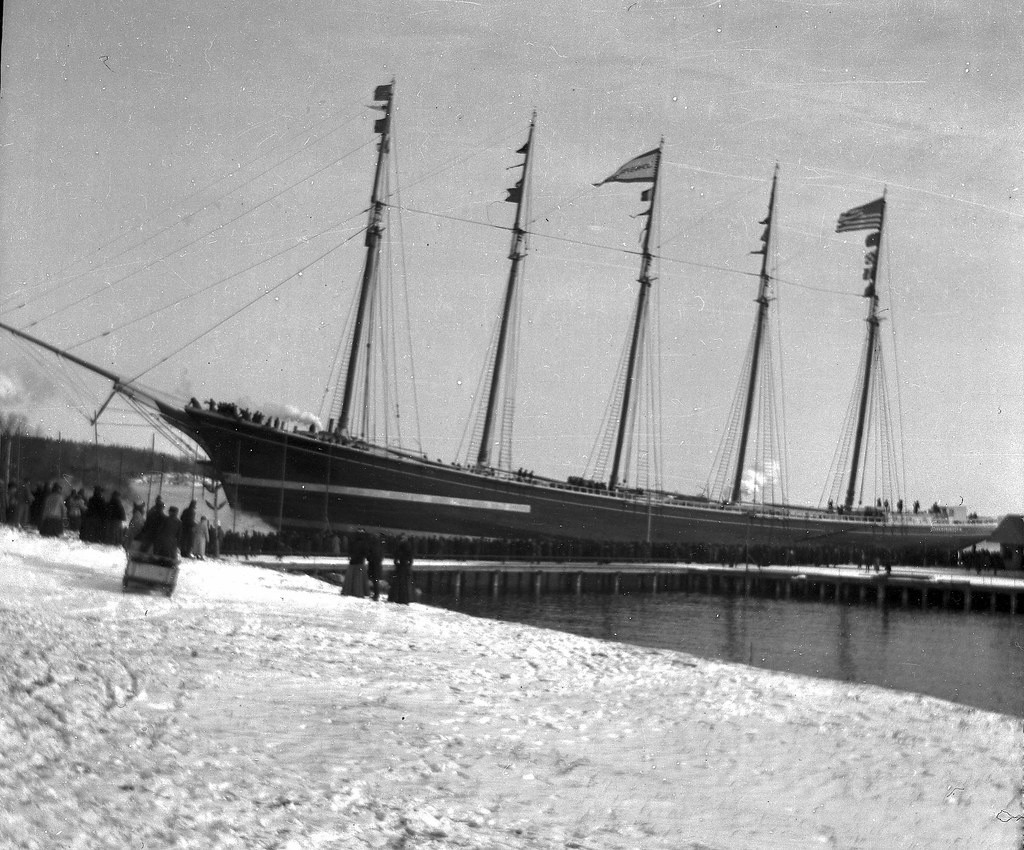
[387,532,415,604]
[186,392,317,437]
[7,477,340,557]
[873,498,945,514]
[181,499,197,558]
[79,486,106,543]
[192,515,209,559]
[162,506,183,568]
[340,526,372,598]
[568,477,605,497]
[417,533,1006,577]
[122,502,144,563]
[39,483,68,538]
[101,491,124,546]
[518,468,533,483]
[139,496,165,563]
[359,533,385,602]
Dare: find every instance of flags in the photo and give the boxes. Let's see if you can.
[593,149,660,187]
[834,200,884,234]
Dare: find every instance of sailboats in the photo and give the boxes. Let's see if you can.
[0,73,1024,566]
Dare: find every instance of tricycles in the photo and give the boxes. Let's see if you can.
[122,536,180,597]
